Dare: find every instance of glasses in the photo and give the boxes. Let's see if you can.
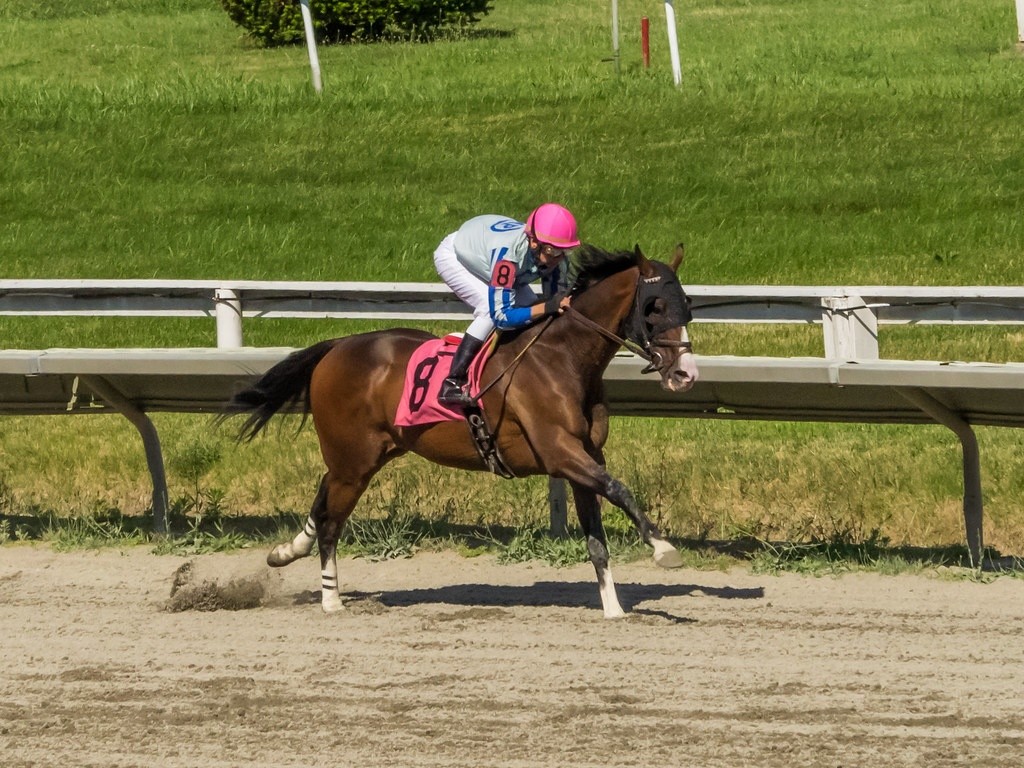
[540,242,574,257]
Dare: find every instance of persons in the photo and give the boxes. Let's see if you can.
[435,202,582,407]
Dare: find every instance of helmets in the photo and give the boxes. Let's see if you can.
[524,203,581,248]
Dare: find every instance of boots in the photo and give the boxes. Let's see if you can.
[437,332,485,408]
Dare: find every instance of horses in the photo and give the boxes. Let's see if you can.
[210,240,702,623]
